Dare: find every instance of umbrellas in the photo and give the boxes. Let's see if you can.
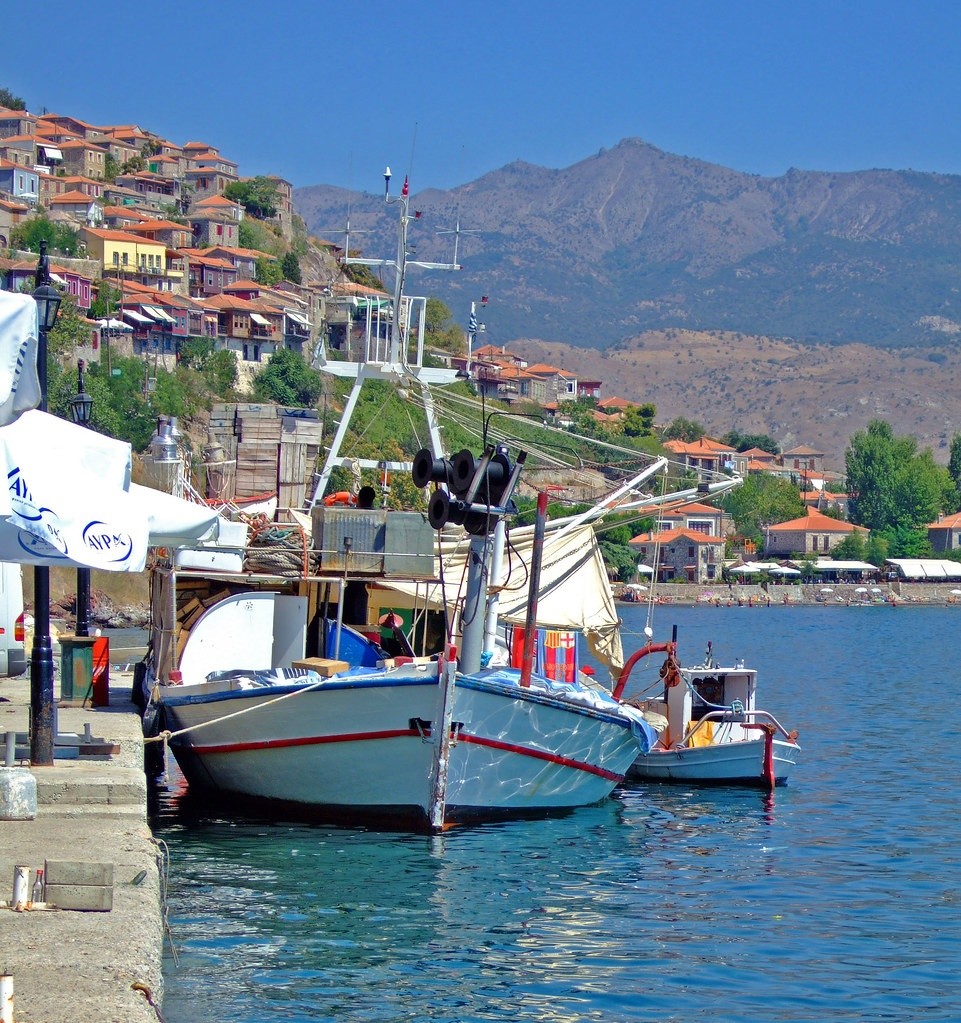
[870,588,881,593]
[729,565,760,582]
[950,589,961,594]
[627,584,647,591]
[820,588,833,593]
[855,587,867,593]
[770,568,800,578]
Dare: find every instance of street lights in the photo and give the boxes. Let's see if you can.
[66,359,93,637]
[22,238,64,767]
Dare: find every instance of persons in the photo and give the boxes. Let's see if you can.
[763,595,770,607]
[748,597,753,607]
[716,598,720,607]
[727,598,732,606]
[887,595,896,607]
[661,649,680,704]
[738,597,744,606]
[622,587,640,599]
[783,593,789,605]
[824,599,828,606]
[708,596,713,604]
[845,597,850,606]
[696,595,700,603]
[357,486,375,509]
[945,595,957,609]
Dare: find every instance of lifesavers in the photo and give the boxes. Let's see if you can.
[325,492,357,507]
[131,661,148,706]
[141,706,167,776]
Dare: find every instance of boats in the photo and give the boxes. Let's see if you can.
[628,640,802,793]
[156,119,665,831]
